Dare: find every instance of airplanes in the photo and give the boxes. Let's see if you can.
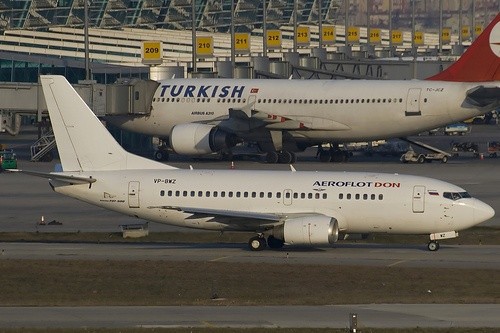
[107,13,500,164]
[4,74,496,252]
